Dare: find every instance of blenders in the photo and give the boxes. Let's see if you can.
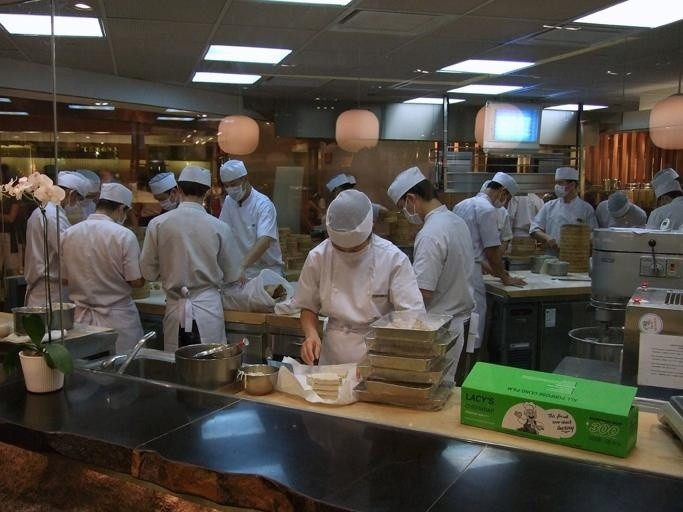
[566,224,682,361]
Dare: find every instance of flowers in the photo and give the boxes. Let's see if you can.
[0,166,73,377]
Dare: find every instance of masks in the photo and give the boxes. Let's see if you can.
[494,198,507,208]
[401,208,424,226]
[554,184,569,197]
[333,244,371,267]
[158,198,177,211]
[65,198,96,222]
[225,185,247,201]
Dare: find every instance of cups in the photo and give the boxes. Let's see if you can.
[601,178,610,193]
[517,154,531,174]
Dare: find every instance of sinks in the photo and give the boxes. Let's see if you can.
[83,349,244,396]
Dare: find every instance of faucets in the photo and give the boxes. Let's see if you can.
[116,330,157,375]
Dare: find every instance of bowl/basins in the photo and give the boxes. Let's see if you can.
[529,255,553,273]
[545,260,569,276]
[40,302,75,331]
[237,364,278,396]
[10,306,49,336]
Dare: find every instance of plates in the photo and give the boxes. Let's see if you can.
[591,185,601,193]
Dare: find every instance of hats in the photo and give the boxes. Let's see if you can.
[607,192,631,217]
[386,165,427,205]
[326,188,374,248]
[325,173,358,189]
[652,167,682,197]
[492,171,520,196]
[99,182,134,208]
[178,166,214,189]
[56,168,100,196]
[554,166,580,182]
[148,171,179,195]
[219,159,248,181]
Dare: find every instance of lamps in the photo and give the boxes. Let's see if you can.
[335,80,379,153]
[474,102,526,157]
[646,71,682,150]
[216,86,259,156]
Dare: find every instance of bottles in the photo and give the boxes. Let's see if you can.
[192,338,250,359]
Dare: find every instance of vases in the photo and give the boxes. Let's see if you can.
[17,351,63,395]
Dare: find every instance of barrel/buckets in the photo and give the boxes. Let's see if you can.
[567,325,623,363]
